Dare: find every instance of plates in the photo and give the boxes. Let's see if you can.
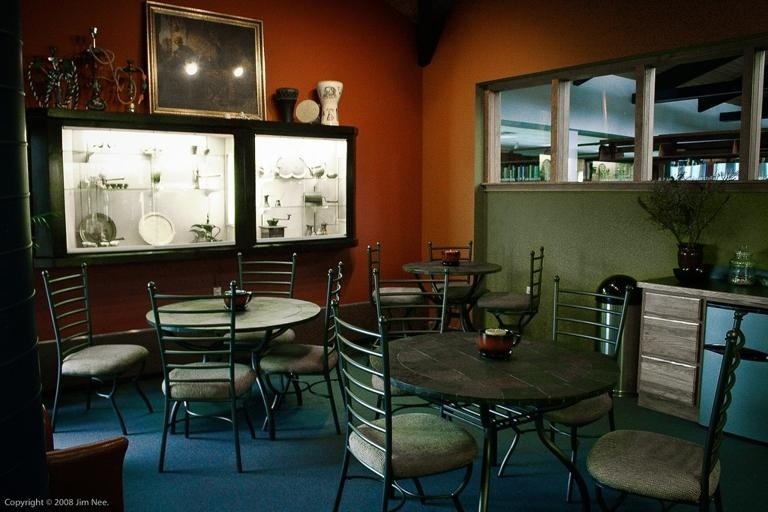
[78,213,117,247]
[138,212,176,245]
[295,99,320,124]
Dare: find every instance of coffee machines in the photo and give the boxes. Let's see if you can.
[260,214,291,239]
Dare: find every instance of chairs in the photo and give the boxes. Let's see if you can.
[330,298,478,512]
[587,310,745,512]
[373,268,452,421]
[223,253,297,402]
[148,281,256,473]
[428,241,472,332]
[367,240,423,367]
[477,247,544,336]
[259,260,347,435]
[497,276,633,503]
[42,262,153,435]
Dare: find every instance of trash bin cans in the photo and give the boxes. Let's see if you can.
[595,275,642,397]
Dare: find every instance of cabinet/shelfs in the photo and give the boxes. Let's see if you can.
[636,284,708,422]
[30,111,244,267]
[246,123,359,254]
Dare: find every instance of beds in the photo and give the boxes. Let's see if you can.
[145,297,322,441]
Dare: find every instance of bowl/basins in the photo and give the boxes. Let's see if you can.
[673,267,712,287]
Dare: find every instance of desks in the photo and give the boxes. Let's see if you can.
[369,333,620,512]
[402,263,501,332]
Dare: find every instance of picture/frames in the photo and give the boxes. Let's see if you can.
[146,1,268,121]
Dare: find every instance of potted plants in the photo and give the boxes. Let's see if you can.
[637,172,742,267]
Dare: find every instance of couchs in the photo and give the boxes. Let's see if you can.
[43,406,129,512]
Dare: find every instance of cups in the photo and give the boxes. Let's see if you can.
[222,290,253,310]
[442,249,461,267]
[477,328,522,359]
